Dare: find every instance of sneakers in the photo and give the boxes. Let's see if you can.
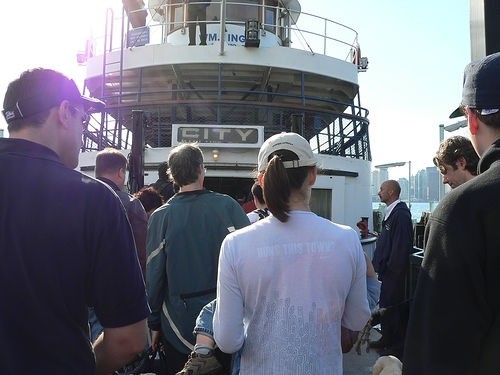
[175,349,222,375]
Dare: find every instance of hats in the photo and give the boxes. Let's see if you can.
[2,68,106,123]
[449,52,500,118]
[258,131,317,171]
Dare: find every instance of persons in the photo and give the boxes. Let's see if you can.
[0,52,500,375]
[184,0,211,46]
[122,0,148,28]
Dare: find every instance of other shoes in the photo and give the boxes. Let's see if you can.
[369,336,391,348]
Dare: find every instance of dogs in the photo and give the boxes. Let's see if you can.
[371,354,402,374]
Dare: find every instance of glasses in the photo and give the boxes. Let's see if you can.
[72,104,90,127]
[439,158,459,174]
